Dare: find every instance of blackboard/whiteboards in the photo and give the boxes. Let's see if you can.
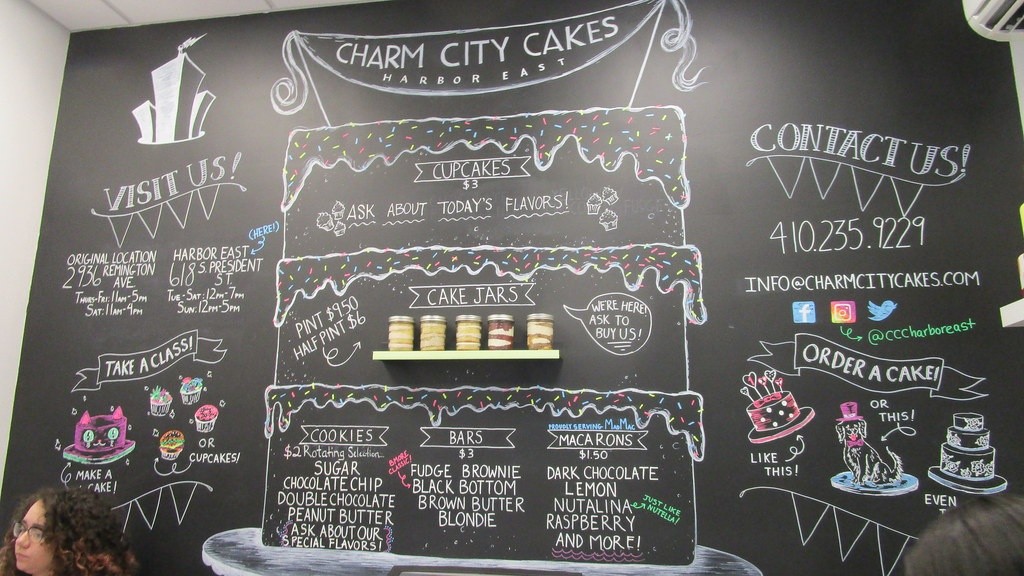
[0,0,1024,576]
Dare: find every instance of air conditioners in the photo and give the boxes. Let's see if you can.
[961,0,1024,43]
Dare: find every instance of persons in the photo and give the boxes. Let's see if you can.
[904,492,1024,576]
[0,486,141,576]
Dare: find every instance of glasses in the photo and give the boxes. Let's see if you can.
[13,521,44,543]
